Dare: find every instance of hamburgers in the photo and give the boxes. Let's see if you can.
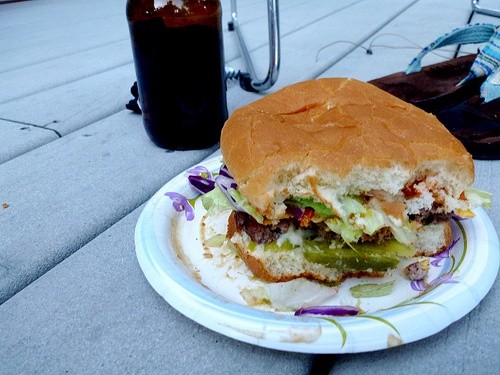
[202,76,491,316]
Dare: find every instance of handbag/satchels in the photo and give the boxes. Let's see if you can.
[368,23,500,162]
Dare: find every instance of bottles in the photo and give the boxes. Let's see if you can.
[124,0,228,153]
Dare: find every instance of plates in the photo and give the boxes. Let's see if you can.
[134,155,500,354]
